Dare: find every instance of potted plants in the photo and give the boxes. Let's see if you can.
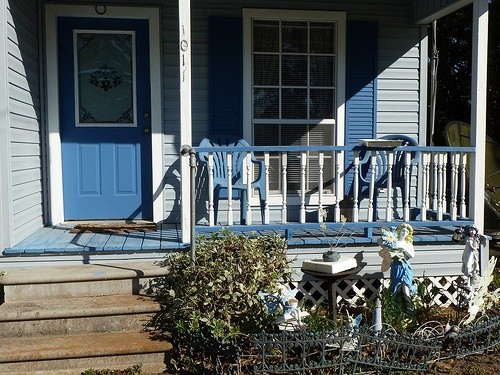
[319,207,352,261]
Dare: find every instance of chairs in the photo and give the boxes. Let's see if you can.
[197,132,268,224]
[350,133,423,222]
